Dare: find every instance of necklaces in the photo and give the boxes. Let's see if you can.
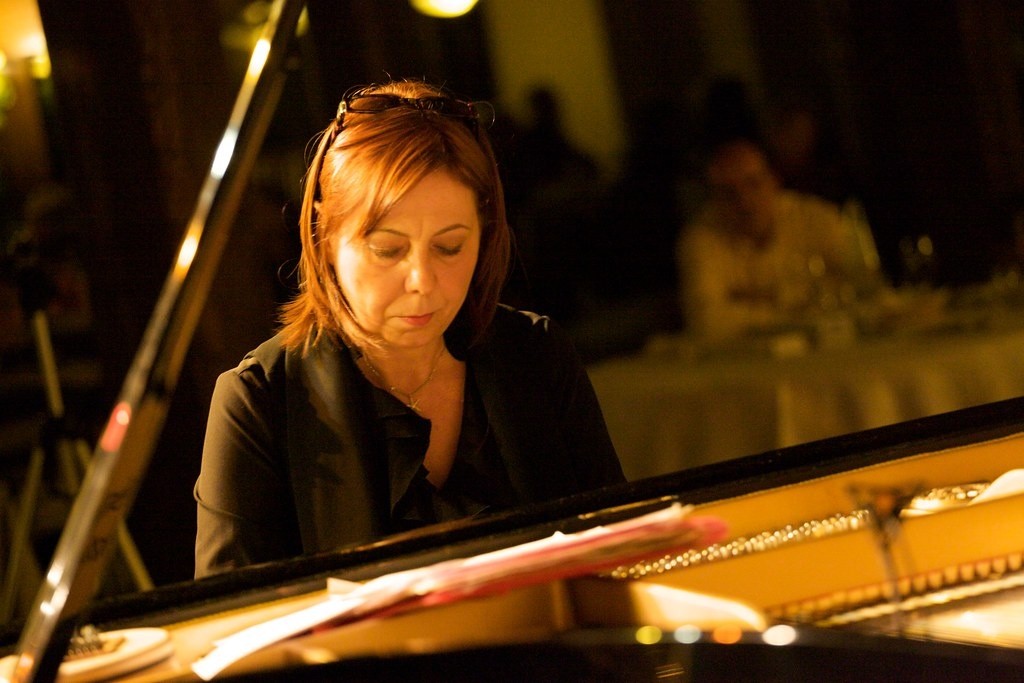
[359,339,445,412]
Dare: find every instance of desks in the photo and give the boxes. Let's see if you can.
[592,293,1024,478]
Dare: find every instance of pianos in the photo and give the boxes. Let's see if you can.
[0,1,1024,683]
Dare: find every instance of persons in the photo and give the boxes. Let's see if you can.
[195,76,627,580]
[677,128,883,339]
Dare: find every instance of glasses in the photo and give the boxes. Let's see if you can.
[329,94,493,153]
